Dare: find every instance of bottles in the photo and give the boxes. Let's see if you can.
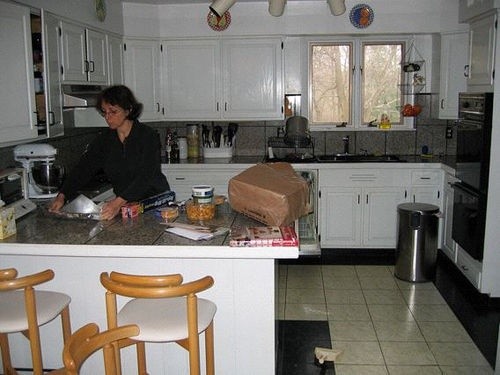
[176,135,189,159]
[187,123,200,159]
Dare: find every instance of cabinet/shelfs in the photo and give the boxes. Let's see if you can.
[315,169,444,251]
[0,0,283,148]
[164,167,235,203]
[437,14,500,120]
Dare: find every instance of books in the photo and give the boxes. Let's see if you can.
[229,225,298,248]
[159,220,230,241]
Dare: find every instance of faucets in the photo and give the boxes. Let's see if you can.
[342,137,350,155]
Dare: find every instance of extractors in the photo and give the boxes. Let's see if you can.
[61,84,107,108]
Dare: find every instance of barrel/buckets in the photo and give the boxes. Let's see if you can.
[284,115,308,143]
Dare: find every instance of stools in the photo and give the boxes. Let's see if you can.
[0,263,218,375]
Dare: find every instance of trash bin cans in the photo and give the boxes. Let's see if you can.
[395,203,440,283]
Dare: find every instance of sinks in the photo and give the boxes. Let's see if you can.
[316,154,400,162]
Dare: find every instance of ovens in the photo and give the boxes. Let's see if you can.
[451,91,494,261]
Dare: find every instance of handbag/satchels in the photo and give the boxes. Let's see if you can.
[227,163,311,228]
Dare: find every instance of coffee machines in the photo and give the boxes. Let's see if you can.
[13,144,65,200]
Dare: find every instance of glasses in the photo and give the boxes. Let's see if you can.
[99,108,122,116]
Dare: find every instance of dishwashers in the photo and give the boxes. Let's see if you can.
[293,170,321,255]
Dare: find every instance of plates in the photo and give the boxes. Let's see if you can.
[207,10,231,30]
[349,4,374,28]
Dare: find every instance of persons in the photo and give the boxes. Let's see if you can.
[47,84,171,222]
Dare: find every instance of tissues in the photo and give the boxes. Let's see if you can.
[0,199,17,239]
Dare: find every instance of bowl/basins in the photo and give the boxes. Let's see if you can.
[32,167,60,192]
